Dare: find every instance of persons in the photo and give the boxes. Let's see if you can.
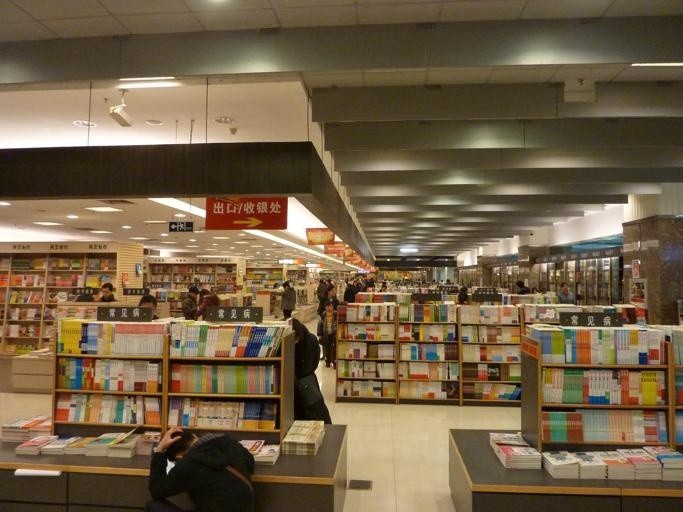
[142,426,256,512]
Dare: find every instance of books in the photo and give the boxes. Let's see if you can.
[0,256,683,482]
